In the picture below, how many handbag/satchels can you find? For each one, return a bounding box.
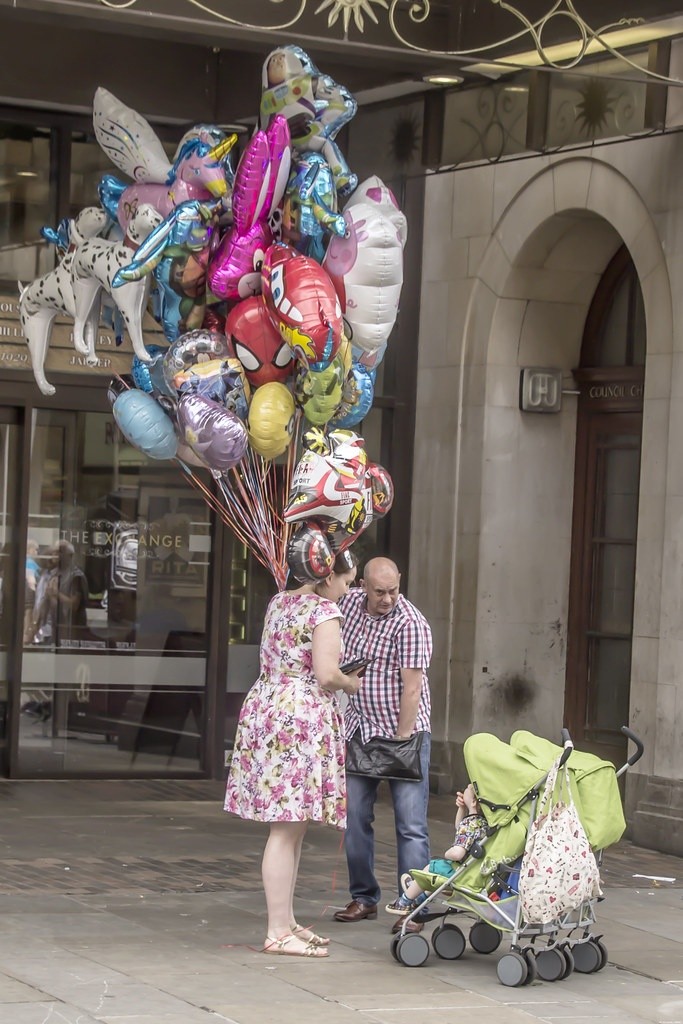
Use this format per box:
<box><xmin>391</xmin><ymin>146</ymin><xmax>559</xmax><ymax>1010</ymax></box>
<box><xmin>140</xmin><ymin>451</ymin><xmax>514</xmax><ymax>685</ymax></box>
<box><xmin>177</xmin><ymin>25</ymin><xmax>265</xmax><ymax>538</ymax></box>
<box><xmin>518</xmin><ymin>802</ymin><xmax>603</xmax><ymax>924</ymax></box>
<box><xmin>345</xmin><ymin>731</ymin><xmax>424</xmax><ymax>783</ymax></box>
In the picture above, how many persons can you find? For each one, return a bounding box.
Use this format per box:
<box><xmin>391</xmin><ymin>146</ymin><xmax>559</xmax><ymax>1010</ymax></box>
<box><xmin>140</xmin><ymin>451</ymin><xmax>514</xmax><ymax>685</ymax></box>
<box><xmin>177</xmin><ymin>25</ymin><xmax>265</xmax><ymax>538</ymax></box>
<box><xmin>334</xmin><ymin>557</ymin><xmax>431</xmax><ymax>933</ymax></box>
<box><xmin>25</xmin><ymin>538</ymin><xmax>88</xmax><ymax>716</ymax></box>
<box><xmin>223</xmin><ymin>549</ymin><xmax>365</xmax><ymax>957</ymax></box>
<box><xmin>385</xmin><ymin>784</ymin><xmax>495</xmax><ymax>914</ymax></box>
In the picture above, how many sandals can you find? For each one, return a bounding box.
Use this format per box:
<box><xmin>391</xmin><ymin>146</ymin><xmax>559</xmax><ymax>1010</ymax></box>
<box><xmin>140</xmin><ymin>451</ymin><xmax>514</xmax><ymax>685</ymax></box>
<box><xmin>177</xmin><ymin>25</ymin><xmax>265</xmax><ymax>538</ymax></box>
<box><xmin>264</xmin><ymin>924</ymin><xmax>332</xmax><ymax>956</ymax></box>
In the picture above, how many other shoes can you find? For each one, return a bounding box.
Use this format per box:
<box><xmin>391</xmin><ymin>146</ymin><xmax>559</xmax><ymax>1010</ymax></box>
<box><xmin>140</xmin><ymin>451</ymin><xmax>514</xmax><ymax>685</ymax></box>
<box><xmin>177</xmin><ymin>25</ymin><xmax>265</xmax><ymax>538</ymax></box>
<box><xmin>400</xmin><ymin>873</ymin><xmax>423</xmax><ymax>908</ymax></box>
<box><xmin>385</xmin><ymin>898</ymin><xmax>413</xmax><ymax>915</ymax></box>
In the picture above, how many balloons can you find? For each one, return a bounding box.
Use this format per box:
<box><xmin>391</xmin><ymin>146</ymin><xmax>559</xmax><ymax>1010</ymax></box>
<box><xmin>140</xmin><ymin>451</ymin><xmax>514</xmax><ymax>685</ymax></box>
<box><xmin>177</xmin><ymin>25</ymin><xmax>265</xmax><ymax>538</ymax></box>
<box><xmin>19</xmin><ymin>48</ymin><xmax>407</xmax><ymax>582</ymax></box>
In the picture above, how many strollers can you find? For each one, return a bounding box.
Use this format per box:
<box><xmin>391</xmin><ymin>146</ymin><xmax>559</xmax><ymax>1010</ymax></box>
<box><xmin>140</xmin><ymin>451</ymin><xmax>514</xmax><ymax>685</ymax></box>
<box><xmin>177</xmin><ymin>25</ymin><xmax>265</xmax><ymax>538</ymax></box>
<box><xmin>385</xmin><ymin>726</ymin><xmax>644</xmax><ymax>988</ymax></box>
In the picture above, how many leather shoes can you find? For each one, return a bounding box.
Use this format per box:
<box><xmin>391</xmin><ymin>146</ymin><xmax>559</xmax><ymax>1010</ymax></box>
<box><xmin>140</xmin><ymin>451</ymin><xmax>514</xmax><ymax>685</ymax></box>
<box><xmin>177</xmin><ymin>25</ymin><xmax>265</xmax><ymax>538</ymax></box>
<box><xmin>392</xmin><ymin>915</ymin><xmax>424</xmax><ymax>932</ymax></box>
<box><xmin>334</xmin><ymin>900</ymin><xmax>377</xmax><ymax>922</ymax></box>
